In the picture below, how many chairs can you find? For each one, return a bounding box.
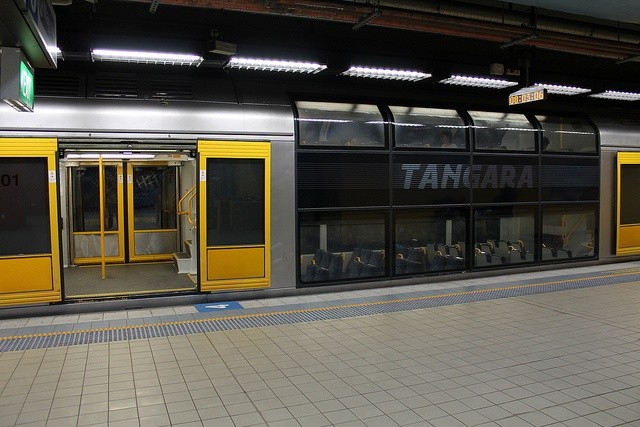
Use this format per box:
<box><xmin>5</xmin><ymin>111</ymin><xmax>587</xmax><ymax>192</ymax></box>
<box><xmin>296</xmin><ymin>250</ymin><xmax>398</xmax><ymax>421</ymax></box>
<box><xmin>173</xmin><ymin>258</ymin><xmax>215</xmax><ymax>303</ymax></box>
<box><xmin>509</xmin><ymin>240</ymin><xmax>525</xmax><ymax>263</ymax></box>
<box><xmin>425</xmin><ymin>242</ymin><xmax>442</xmax><ymax>272</ymax></box>
<box><xmin>303</xmin><ymin>250</ymin><xmax>343</xmax><ymax>282</ymax></box>
<box><xmin>498</xmin><ymin>240</ymin><xmax>507</xmax><ymax>256</ymax></box>
<box><xmin>475</xmin><ymin>241</ymin><xmax>494</xmax><ymax>268</ymax></box>
<box><xmin>347</xmin><ymin>247</ymin><xmax>384</xmax><ymax>279</ymax></box>
<box><xmin>394</xmin><ymin>254</ymin><xmax>425</xmax><ymax>274</ymax></box>
<box><xmin>443</xmin><ymin>243</ymin><xmax>465</xmax><ymax>272</ymax></box>
<box><xmin>542</xmin><ymin>247</ymin><xmax>571</xmax><ymax>260</ymax></box>
